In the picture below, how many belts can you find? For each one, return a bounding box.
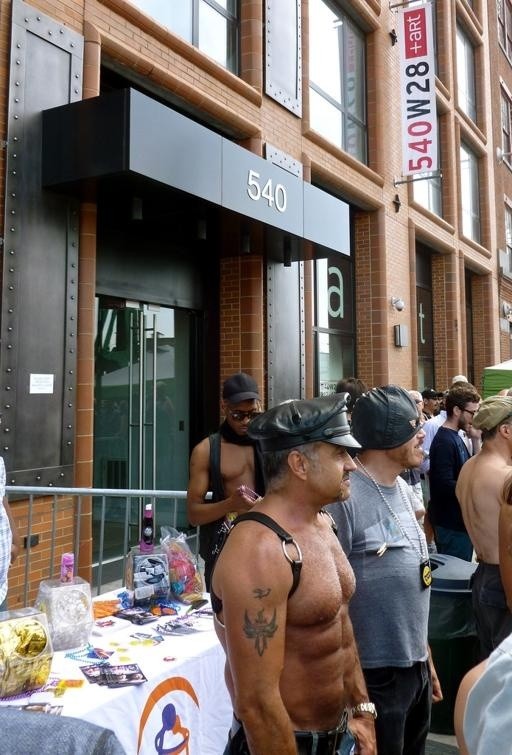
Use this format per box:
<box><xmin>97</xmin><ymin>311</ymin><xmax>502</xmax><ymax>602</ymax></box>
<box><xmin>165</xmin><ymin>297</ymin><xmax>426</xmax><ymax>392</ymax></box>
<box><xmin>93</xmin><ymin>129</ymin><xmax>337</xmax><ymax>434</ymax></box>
<box><xmin>233</xmin><ymin>708</ymin><xmax>348</xmax><ymax>755</ymax></box>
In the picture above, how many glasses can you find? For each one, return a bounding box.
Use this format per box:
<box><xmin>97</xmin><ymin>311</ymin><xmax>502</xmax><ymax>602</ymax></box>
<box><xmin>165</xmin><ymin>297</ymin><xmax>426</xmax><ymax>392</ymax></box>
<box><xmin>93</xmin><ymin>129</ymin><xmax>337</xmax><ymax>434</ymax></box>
<box><xmin>225</xmin><ymin>405</ymin><xmax>261</xmax><ymax>421</ymax></box>
<box><xmin>458</xmin><ymin>408</ymin><xmax>476</xmax><ymax>416</ymax></box>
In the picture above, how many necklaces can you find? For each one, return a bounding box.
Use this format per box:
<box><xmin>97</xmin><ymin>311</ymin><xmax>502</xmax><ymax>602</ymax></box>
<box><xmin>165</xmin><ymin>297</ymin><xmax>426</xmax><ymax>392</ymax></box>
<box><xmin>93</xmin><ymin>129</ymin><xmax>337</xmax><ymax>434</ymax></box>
<box><xmin>355</xmin><ymin>456</ymin><xmax>428</xmax><ymax>560</ymax></box>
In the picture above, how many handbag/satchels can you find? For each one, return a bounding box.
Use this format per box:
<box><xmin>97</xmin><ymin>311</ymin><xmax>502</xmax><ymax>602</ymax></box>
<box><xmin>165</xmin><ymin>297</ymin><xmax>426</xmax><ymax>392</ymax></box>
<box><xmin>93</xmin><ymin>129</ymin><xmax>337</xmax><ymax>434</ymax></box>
<box><xmin>160</xmin><ymin>525</ymin><xmax>205</xmax><ymax>605</ymax></box>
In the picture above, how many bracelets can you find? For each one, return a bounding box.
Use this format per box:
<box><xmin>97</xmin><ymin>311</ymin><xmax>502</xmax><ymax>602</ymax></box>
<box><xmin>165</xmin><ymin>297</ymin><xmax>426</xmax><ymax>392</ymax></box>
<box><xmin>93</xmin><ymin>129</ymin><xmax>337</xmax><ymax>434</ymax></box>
<box><xmin>351</xmin><ymin>702</ymin><xmax>378</xmax><ymax>719</ymax></box>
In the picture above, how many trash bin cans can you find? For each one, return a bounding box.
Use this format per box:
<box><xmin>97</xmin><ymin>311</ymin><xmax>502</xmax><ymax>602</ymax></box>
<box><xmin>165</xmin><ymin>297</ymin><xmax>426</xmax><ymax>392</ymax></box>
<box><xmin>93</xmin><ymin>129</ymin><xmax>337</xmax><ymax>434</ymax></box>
<box><xmin>428</xmin><ymin>552</ymin><xmax>483</xmax><ymax>736</ymax></box>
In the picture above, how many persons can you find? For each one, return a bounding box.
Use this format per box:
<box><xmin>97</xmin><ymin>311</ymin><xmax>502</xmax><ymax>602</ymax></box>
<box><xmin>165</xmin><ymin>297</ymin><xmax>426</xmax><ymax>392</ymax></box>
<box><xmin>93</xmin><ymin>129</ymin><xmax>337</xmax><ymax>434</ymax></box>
<box><xmin>187</xmin><ymin>373</ymin><xmax>266</xmax><ymax>592</ymax></box>
<box><xmin>322</xmin><ymin>385</ymin><xmax>444</xmax><ymax>755</ymax></box>
<box><xmin>116</xmin><ymin>674</ymin><xmax>143</xmax><ymax>683</ymax></box>
<box><xmin>336</xmin><ymin>377</ymin><xmax>368</xmax><ymax>424</ymax></box>
<box><xmin>407</xmin><ymin>375</ymin><xmax>512</xmax><ymax>755</ymax></box>
<box><xmin>0</xmin><ymin>456</ymin><xmax>19</xmax><ymax>612</ymax></box>
<box><xmin>210</xmin><ymin>391</ymin><xmax>379</xmax><ymax>755</ymax></box>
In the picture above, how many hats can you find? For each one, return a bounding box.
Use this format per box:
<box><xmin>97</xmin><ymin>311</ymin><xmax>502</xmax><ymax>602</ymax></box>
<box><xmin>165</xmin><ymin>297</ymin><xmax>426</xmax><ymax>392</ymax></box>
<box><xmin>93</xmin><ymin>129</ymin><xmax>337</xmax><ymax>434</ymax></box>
<box><xmin>421</xmin><ymin>388</ymin><xmax>443</xmax><ymax>397</ymax></box>
<box><xmin>352</xmin><ymin>384</ymin><xmax>423</xmax><ymax>449</ymax></box>
<box><xmin>473</xmin><ymin>396</ymin><xmax>511</xmax><ymax>432</ymax></box>
<box><xmin>222</xmin><ymin>373</ymin><xmax>259</xmax><ymax>404</ymax></box>
<box><xmin>247</xmin><ymin>392</ymin><xmax>363</xmax><ymax>450</ymax></box>
